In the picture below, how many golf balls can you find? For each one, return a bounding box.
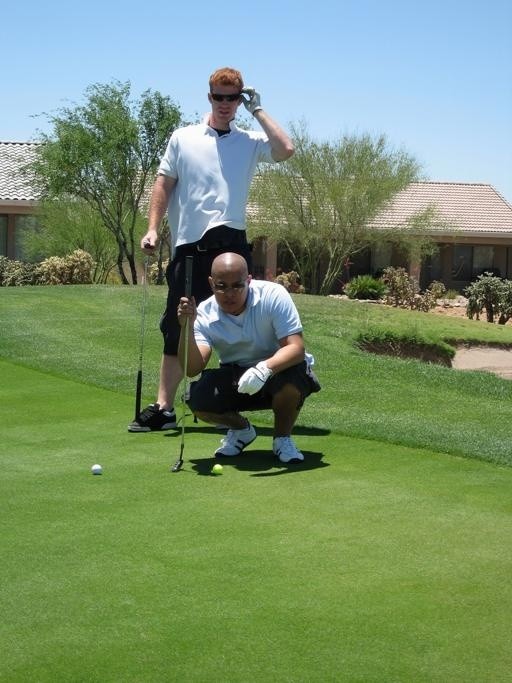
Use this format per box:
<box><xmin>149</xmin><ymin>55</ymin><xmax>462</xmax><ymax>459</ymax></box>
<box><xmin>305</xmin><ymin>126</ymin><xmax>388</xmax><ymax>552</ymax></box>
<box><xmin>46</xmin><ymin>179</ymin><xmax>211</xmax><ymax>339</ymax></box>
<box><xmin>212</xmin><ymin>464</ymin><xmax>224</xmax><ymax>475</ymax></box>
<box><xmin>91</xmin><ymin>463</ymin><xmax>101</xmax><ymax>473</ymax></box>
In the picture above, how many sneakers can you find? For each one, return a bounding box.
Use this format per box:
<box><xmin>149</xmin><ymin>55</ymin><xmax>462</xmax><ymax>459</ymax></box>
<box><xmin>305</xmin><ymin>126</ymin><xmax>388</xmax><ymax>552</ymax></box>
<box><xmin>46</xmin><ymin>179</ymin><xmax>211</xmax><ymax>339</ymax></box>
<box><xmin>214</xmin><ymin>418</ymin><xmax>257</xmax><ymax>457</ymax></box>
<box><xmin>128</xmin><ymin>402</ymin><xmax>177</xmax><ymax>432</ymax></box>
<box><xmin>273</xmin><ymin>436</ymin><xmax>305</xmax><ymax>464</ymax></box>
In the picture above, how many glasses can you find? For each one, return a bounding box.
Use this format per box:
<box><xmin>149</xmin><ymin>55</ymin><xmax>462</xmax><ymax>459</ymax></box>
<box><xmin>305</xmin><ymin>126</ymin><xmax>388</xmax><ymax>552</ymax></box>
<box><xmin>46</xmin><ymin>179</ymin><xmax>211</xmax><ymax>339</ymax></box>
<box><xmin>211</xmin><ymin>278</ymin><xmax>249</xmax><ymax>294</ymax></box>
<box><xmin>210</xmin><ymin>90</ymin><xmax>241</xmax><ymax>101</ymax></box>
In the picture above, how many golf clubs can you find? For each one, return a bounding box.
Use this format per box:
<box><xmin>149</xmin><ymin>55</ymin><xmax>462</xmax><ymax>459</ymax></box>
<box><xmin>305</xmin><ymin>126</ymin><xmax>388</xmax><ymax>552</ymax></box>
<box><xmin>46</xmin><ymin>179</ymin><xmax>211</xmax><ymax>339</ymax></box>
<box><xmin>135</xmin><ymin>240</ymin><xmax>149</xmax><ymax>417</ymax></box>
<box><xmin>172</xmin><ymin>255</ymin><xmax>193</xmax><ymax>474</ymax></box>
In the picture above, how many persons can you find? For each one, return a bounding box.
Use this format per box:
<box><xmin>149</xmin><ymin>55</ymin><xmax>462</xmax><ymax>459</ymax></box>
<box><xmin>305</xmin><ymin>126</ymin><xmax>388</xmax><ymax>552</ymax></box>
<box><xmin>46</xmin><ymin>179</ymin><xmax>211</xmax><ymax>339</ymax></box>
<box><xmin>125</xmin><ymin>66</ymin><xmax>296</xmax><ymax>432</ymax></box>
<box><xmin>178</xmin><ymin>251</ymin><xmax>321</xmax><ymax>464</ymax></box>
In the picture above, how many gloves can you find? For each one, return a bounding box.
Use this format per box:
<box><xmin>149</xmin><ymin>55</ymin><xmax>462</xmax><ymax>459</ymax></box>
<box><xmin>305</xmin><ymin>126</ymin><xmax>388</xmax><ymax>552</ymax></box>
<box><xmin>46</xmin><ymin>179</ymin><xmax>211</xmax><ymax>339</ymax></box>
<box><xmin>237</xmin><ymin>361</ymin><xmax>273</xmax><ymax>395</ymax></box>
<box><xmin>241</xmin><ymin>87</ymin><xmax>264</xmax><ymax>116</ymax></box>
